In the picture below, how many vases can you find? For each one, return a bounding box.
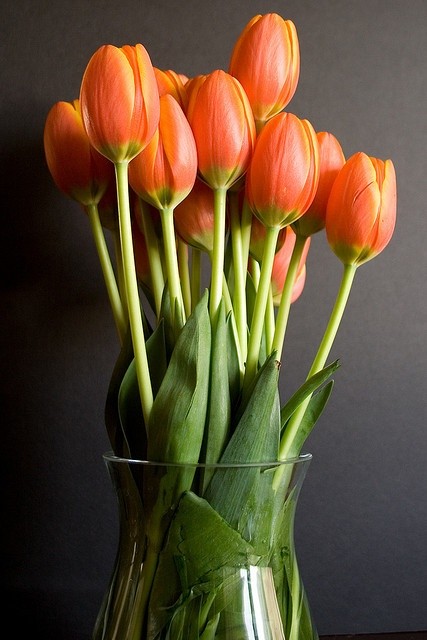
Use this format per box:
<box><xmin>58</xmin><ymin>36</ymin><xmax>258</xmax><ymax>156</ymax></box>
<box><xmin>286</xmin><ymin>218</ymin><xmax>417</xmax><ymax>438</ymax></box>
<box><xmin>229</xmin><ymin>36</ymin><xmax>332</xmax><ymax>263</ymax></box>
<box><xmin>90</xmin><ymin>451</ymin><xmax>320</xmax><ymax>640</ymax></box>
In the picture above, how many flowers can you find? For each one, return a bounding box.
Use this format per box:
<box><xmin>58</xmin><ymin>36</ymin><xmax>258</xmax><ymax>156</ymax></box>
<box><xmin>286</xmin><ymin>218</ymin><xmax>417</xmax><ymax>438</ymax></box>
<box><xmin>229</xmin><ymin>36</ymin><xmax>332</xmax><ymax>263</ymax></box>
<box><xmin>43</xmin><ymin>12</ymin><xmax>397</xmax><ymax>640</ymax></box>
